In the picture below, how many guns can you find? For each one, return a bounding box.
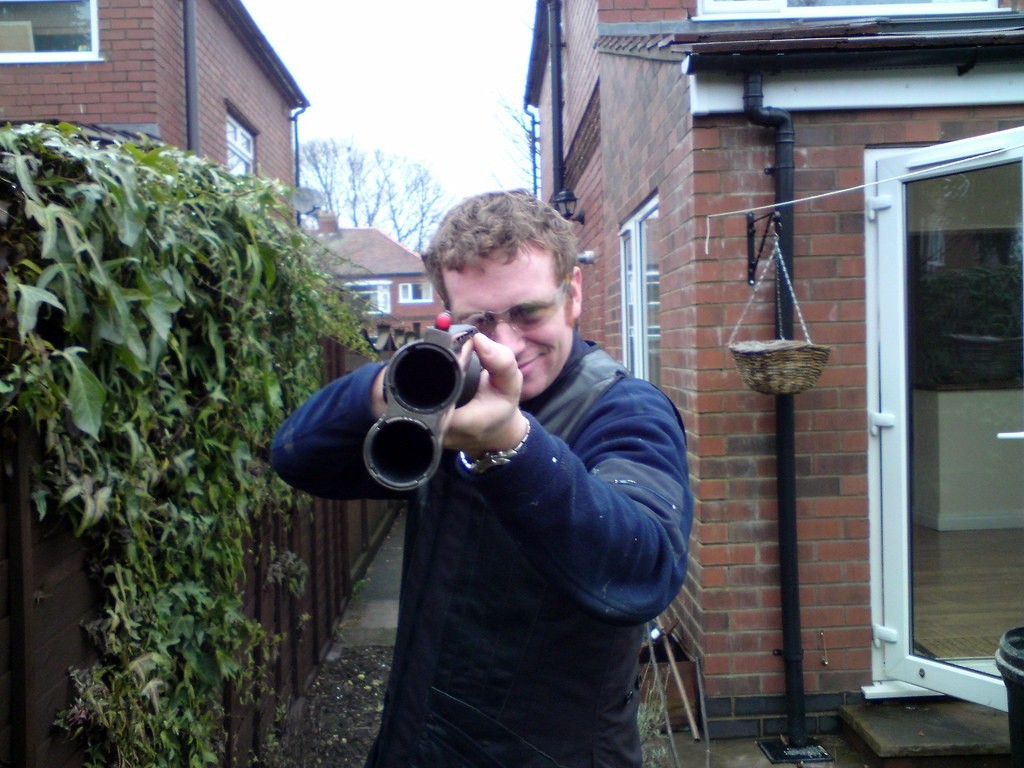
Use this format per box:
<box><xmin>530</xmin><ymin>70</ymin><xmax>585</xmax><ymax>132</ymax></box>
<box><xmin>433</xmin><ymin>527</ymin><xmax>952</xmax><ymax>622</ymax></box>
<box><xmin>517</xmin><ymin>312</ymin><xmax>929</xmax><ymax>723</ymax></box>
<box><xmin>365</xmin><ymin>315</ymin><xmax>483</xmax><ymax>488</ymax></box>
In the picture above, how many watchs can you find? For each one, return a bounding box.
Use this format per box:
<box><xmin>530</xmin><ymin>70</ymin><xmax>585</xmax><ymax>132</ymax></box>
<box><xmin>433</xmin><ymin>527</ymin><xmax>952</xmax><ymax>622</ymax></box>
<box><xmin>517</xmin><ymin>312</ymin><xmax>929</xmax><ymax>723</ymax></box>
<box><xmin>458</xmin><ymin>417</ymin><xmax>530</xmax><ymax>473</ymax></box>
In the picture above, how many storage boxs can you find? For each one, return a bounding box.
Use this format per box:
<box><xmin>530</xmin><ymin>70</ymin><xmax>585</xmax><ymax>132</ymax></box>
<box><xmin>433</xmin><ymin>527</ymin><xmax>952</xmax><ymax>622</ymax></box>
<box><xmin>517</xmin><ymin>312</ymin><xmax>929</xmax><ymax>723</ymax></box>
<box><xmin>638</xmin><ymin>616</ymin><xmax>699</xmax><ymax>733</ymax></box>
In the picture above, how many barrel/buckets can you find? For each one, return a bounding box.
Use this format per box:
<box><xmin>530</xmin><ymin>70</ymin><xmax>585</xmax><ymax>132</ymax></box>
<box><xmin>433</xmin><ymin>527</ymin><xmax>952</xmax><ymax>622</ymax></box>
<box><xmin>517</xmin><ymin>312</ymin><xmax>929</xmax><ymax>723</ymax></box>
<box><xmin>994</xmin><ymin>625</ymin><xmax>1024</xmax><ymax>768</ymax></box>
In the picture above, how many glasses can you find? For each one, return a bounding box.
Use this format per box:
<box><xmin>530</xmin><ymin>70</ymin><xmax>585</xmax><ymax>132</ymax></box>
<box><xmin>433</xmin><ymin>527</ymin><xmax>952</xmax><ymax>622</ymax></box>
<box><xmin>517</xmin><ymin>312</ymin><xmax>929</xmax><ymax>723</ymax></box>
<box><xmin>445</xmin><ymin>279</ymin><xmax>567</xmax><ymax>341</ymax></box>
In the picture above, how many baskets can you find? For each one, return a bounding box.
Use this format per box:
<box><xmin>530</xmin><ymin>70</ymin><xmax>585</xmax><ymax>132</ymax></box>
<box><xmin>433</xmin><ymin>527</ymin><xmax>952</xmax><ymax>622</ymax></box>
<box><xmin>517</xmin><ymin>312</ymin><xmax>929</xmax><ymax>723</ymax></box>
<box><xmin>728</xmin><ymin>341</ymin><xmax>831</xmax><ymax>395</ymax></box>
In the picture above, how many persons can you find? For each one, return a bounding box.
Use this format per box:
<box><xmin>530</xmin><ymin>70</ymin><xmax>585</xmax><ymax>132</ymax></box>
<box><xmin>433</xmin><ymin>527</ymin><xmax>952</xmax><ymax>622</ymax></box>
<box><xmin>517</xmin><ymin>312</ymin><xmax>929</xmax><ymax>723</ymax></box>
<box><xmin>269</xmin><ymin>189</ymin><xmax>697</xmax><ymax>768</ymax></box>
